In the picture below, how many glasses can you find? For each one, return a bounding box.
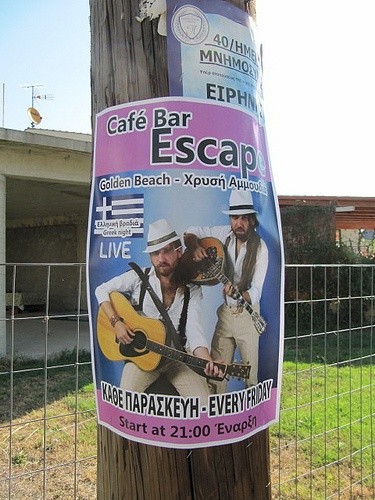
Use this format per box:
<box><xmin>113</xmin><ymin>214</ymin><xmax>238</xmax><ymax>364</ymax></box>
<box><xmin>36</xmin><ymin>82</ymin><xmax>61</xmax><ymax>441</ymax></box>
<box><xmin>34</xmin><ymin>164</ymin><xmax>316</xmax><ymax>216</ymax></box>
<box><xmin>149</xmin><ymin>246</ymin><xmax>182</xmax><ymax>256</ymax></box>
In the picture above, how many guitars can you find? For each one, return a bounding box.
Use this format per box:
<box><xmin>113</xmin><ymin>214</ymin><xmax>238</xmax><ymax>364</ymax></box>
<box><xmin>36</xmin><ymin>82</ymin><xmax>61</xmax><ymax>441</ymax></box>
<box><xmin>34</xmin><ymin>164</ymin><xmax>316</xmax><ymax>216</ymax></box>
<box><xmin>180</xmin><ymin>236</ymin><xmax>267</xmax><ymax>336</ymax></box>
<box><xmin>96</xmin><ymin>291</ymin><xmax>251</xmax><ymax>381</ymax></box>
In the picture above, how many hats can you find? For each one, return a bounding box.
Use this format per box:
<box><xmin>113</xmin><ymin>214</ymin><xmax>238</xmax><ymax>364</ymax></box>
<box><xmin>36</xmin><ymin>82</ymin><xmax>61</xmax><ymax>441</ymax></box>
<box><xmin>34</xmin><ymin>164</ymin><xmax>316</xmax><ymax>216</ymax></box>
<box><xmin>222</xmin><ymin>190</ymin><xmax>258</xmax><ymax>216</ymax></box>
<box><xmin>141</xmin><ymin>219</ymin><xmax>183</xmax><ymax>253</ymax></box>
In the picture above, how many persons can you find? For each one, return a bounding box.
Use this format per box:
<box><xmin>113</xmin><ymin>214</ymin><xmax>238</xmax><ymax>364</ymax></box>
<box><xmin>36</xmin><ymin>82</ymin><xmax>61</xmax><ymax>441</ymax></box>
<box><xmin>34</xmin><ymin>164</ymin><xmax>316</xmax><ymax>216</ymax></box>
<box><xmin>95</xmin><ymin>219</ymin><xmax>226</xmax><ymax>413</ymax></box>
<box><xmin>184</xmin><ymin>189</ymin><xmax>269</xmax><ymax>396</ymax></box>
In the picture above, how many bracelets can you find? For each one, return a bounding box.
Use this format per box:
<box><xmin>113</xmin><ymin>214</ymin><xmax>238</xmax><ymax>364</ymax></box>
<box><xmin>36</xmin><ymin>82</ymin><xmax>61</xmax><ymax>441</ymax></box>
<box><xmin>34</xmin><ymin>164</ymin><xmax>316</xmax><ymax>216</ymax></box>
<box><xmin>108</xmin><ymin>313</ymin><xmax>121</xmax><ymax>327</ymax></box>
